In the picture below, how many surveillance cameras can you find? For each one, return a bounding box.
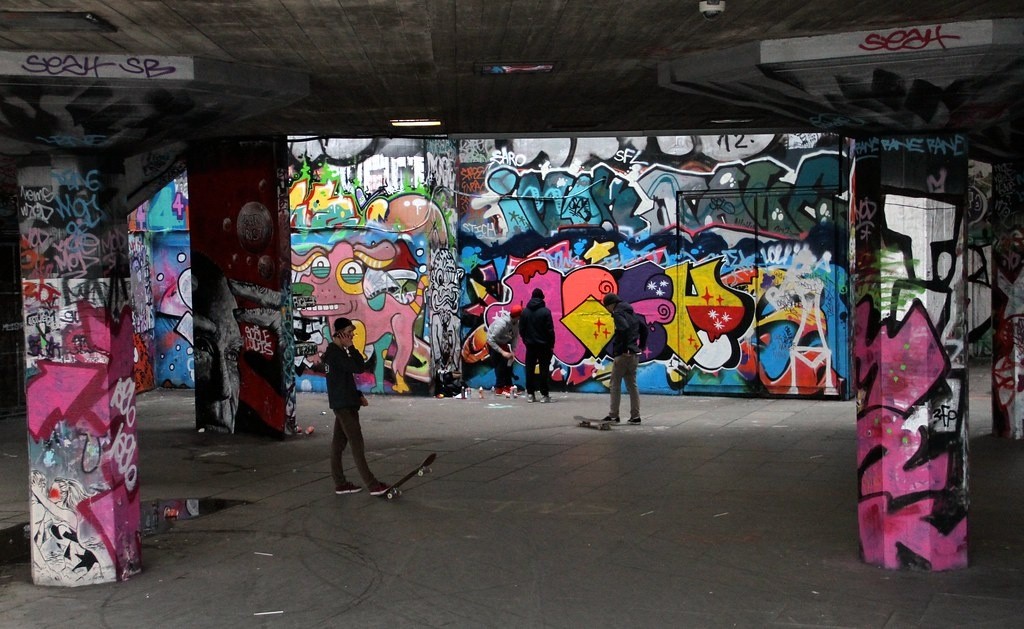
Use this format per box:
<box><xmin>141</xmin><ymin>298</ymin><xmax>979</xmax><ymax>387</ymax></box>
<box><xmin>699</xmin><ymin>0</ymin><xmax>726</xmax><ymax>21</ymax></box>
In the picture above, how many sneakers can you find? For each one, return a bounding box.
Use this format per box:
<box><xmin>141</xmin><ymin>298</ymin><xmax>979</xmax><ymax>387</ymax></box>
<box><xmin>527</xmin><ymin>395</ymin><xmax>536</xmax><ymax>403</ymax></box>
<box><xmin>368</xmin><ymin>477</ymin><xmax>391</xmax><ymax>495</ymax></box>
<box><xmin>540</xmin><ymin>394</ymin><xmax>551</xmax><ymax>402</ymax></box>
<box><xmin>496</xmin><ymin>387</ymin><xmax>518</xmax><ymax>398</ymax></box>
<box><xmin>602</xmin><ymin>415</ymin><xmax>620</xmax><ymax>422</ymax></box>
<box><xmin>627</xmin><ymin>416</ymin><xmax>641</xmax><ymax>424</ymax></box>
<box><xmin>335</xmin><ymin>480</ymin><xmax>363</xmax><ymax>494</ymax></box>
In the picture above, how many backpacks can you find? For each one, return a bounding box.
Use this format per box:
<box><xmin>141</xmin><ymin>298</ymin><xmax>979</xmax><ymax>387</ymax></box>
<box><xmin>434</xmin><ymin>370</ymin><xmax>466</xmax><ymax>397</ymax></box>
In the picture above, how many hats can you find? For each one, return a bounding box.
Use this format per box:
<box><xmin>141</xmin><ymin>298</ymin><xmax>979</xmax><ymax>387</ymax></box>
<box><xmin>531</xmin><ymin>287</ymin><xmax>545</xmax><ymax>299</ymax></box>
<box><xmin>334</xmin><ymin>317</ymin><xmax>352</xmax><ymax>331</ymax></box>
<box><xmin>603</xmin><ymin>292</ymin><xmax>622</xmax><ymax>306</ymax></box>
<box><xmin>510</xmin><ymin>303</ymin><xmax>524</xmax><ymax>314</ymax></box>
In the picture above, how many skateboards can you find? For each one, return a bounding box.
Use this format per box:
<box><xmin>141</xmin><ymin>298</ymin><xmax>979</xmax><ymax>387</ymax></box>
<box><xmin>380</xmin><ymin>451</ymin><xmax>437</xmax><ymax>499</ymax></box>
<box><xmin>573</xmin><ymin>414</ymin><xmax>619</xmax><ymax>429</ymax></box>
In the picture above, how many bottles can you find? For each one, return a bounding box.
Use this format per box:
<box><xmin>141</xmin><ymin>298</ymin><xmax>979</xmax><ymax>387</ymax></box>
<box><xmin>510</xmin><ymin>387</ymin><xmax>514</xmax><ymax>399</ymax></box>
<box><xmin>467</xmin><ymin>387</ymin><xmax>471</xmax><ymax>399</ymax></box>
<box><xmin>461</xmin><ymin>386</ymin><xmax>465</xmax><ymax>398</ymax></box>
<box><xmin>491</xmin><ymin>385</ymin><xmax>495</xmax><ymax>394</ymax></box>
<box><xmin>479</xmin><ymin>385</ymin><xmax>484</xmax><ymax>399</ymax></box>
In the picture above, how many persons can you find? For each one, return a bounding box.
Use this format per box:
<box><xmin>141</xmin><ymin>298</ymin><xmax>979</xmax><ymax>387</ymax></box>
<box><xmin>602</xmin><ymin>292</ymin><xmax>648</xmax><ymax>425</ymax></box>
<box><xmin>319</xmin><ymin>317</ymin><xmax>392</xmax><ymax>495</ymax></box>
<box><xmin>485</xmin><ymin>305</ymin><xmax>524</xmax><ymax>397</ymax></box>
<box><xmin>520</xmin><ymin>287</ymin><xmax>558</xmax><ymax>403</ymax></box>
